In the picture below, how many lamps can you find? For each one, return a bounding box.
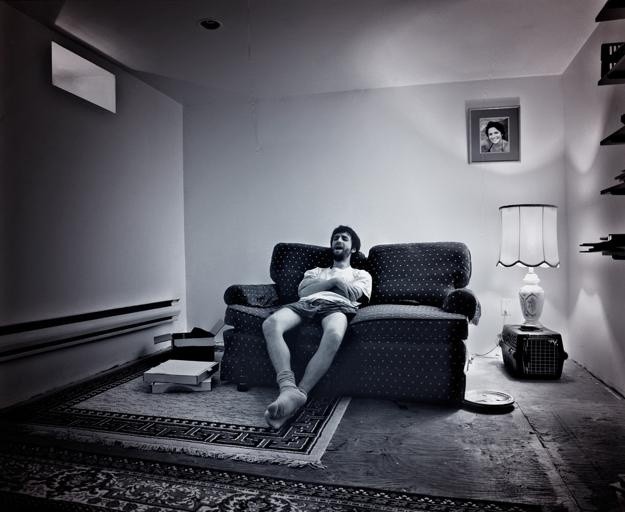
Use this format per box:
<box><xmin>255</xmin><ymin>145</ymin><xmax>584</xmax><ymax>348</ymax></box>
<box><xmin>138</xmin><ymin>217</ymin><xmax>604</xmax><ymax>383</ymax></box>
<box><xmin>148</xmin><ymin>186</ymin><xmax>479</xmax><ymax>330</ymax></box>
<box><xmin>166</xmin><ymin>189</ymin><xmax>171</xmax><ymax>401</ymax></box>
<box><xmin>494</xmin><ymin>202</ymin><xmax>562</xmax><ymax>332</ymax></box>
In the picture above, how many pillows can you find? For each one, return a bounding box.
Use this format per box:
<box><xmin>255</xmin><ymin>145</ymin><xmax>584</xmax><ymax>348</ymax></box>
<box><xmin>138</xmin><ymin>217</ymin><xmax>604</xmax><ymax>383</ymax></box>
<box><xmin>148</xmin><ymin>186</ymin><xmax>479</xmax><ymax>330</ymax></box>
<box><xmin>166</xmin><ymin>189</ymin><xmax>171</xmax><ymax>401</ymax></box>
<box><xmin>367</xmin><ymin>242</ymin><xmax>473</xmax><ymax>307</ymax></box>
<box><xmin>269</xmin><ymin>242</ymin><xmax>367</xmax><ymax>304</ymax></box>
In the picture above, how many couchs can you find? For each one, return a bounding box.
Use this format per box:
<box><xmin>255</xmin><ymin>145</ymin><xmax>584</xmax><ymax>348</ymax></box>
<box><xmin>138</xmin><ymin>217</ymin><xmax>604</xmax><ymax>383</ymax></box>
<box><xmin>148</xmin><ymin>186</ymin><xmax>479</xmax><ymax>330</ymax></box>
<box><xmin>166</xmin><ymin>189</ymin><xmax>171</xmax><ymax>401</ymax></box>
<box><xmin>220</xmin><ymin>241</ymin><xmax>482</xmax><ymax>411</ymax></box>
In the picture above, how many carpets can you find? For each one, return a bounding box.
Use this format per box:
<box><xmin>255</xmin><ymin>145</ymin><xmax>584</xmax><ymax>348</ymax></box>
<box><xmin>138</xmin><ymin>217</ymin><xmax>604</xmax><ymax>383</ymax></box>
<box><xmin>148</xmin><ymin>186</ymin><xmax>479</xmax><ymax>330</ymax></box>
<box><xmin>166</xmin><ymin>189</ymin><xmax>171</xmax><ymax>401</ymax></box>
<box><xmin>0</xmin><ymin>439</ymin><xmax>570</xmax><ymax>512</ymax></box>
<box><xmin>1</xmin><ymin>346</ymin><xmax>351</xmax><ymax>470</ymax></box>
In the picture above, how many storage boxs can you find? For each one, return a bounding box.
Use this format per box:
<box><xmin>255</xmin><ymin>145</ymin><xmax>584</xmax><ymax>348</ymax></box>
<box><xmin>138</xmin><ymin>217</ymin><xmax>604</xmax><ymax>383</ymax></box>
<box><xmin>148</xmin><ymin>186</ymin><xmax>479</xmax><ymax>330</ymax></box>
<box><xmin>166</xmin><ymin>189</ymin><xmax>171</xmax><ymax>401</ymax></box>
<box><xmin>153</xmin><ymin>318</ymin><xmax>226</xmax><ymax>362</ymax></box>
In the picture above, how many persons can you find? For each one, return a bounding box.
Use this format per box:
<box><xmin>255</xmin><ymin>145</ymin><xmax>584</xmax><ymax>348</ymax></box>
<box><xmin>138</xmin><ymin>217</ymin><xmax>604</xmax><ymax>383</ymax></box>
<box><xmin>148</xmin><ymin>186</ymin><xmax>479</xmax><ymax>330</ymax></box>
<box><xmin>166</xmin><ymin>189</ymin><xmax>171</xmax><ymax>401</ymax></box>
<box><xmin>485</xmin><ymin>121</ymin><xmax>509</xmax><ymax>153</ymax></box>
<box><xmin>260</xmin><ymin>225</ymin><xmax>375</xmax><ymax>431</ymax></box>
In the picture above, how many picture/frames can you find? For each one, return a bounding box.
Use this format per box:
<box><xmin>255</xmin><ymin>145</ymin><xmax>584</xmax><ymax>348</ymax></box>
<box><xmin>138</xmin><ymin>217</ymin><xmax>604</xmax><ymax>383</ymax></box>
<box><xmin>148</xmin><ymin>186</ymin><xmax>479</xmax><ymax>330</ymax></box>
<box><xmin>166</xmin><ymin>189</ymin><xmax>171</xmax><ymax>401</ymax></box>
<box><xmin>466</xmin><ymin>104</ymin><xmax>521</xmax><ymax>165</ymax></box>
